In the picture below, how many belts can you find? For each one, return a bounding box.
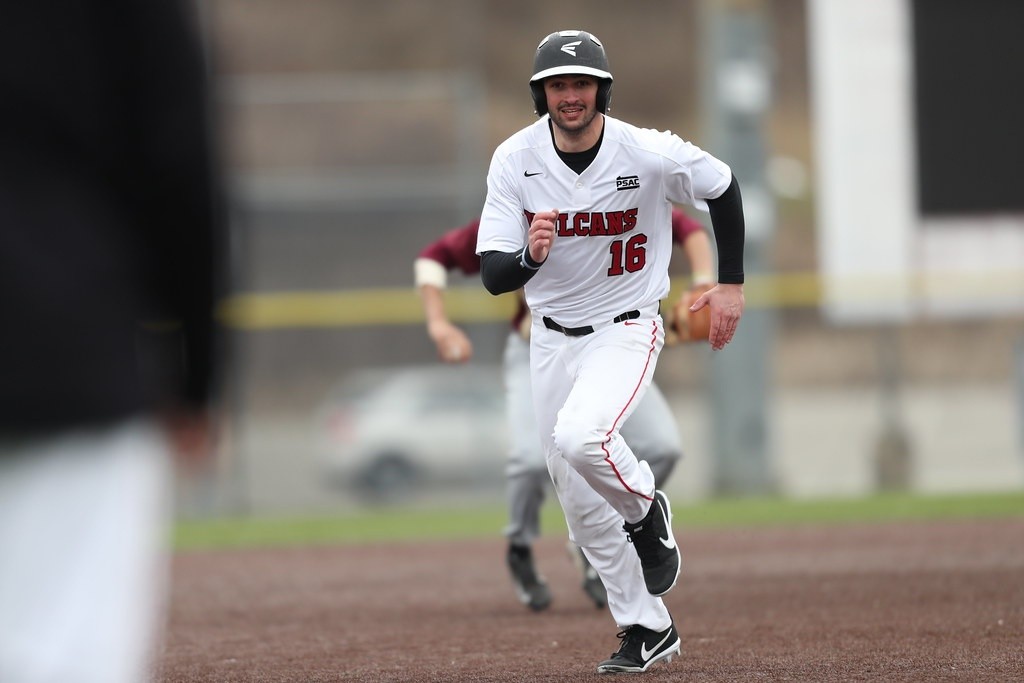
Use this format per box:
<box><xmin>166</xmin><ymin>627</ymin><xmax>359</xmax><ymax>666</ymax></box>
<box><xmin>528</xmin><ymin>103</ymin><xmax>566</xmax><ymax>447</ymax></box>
<box><xmin>543</xmin><ymin>310</ymin><xmax>639</xmax><ymax>337</ymax></box>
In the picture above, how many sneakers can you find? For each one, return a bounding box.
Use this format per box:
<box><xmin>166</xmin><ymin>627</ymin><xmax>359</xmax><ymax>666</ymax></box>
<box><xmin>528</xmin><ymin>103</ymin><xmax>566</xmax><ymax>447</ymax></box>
<box><xmin>621</xmin><ymin>489</ymin><xmax>681</xmax><ymax>597</ymax></box>
<box><xmin>506</xmin><ymin>542</ymin><xmax>552</xmax><ymax>611</ymax></box>
<box><xmin>596</xmin><ymin>612</ymin><xmax>681</xmax><ymax>675</ymax></box>
<box><xmin>565</xmin><ymin>539</ymin><xmax>607</xmax><ymax>607</ymax></box>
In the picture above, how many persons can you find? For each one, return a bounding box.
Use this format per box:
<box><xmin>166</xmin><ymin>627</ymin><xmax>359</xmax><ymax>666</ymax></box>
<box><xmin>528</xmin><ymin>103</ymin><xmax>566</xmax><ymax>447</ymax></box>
<box><xmin>0</xmin><ymin>0</ymin><xmax>229</xmax><ymax>683</ymax></box>
<box><xmin>415</xmin><ymin>29</ymin><xmax>743</xmax><ymax>674</ymax></box>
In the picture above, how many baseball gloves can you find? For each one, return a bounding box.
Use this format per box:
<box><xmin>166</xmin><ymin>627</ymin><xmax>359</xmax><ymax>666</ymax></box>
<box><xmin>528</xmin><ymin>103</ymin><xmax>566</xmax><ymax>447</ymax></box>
<box><xmin>659</xmin><ymin>285</ymin><xmax>717</xmax><ymax>344</ymax></box>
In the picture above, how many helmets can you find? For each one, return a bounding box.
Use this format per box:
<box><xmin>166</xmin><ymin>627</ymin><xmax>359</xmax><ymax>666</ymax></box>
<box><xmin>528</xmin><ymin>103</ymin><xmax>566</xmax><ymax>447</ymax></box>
<box><xmin>529</xmin><ymin>29</ymin><xmax>613</xmax><ymax>118</ymax></box>
